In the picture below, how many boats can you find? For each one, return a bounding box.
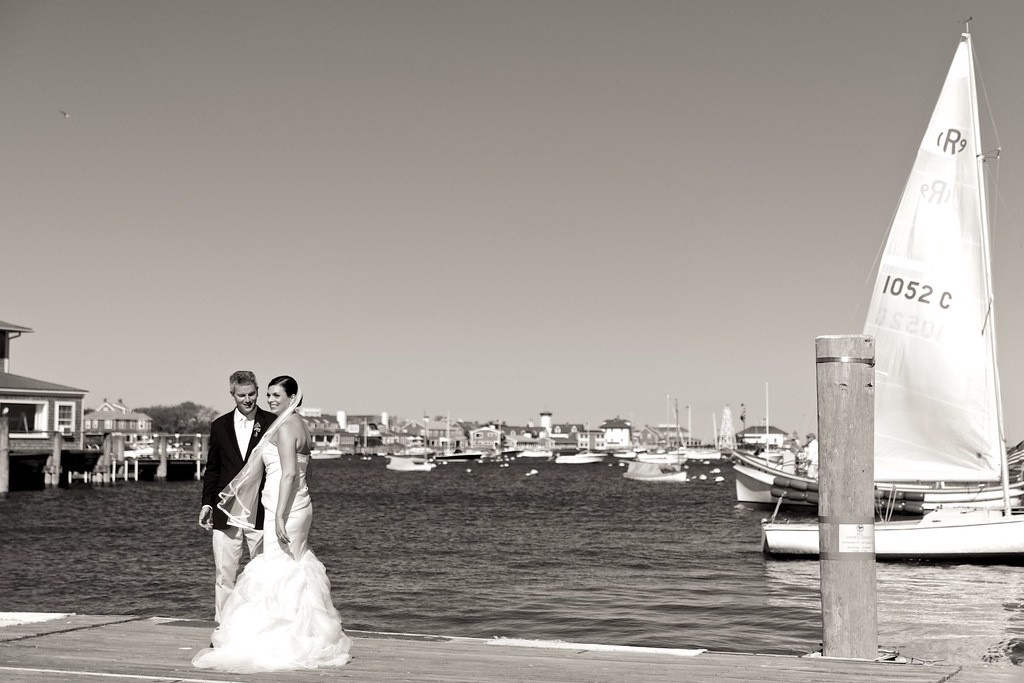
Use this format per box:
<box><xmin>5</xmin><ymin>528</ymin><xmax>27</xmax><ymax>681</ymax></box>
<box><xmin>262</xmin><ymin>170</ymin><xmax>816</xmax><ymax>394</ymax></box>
<box><xmin>386</xmin><ymin>381</ymin><xmax>1024</xmax><ymax>515</ymax></box>
<box><xmin>310</xmin><ymin>447</ymin><xmax>342</xmax><ymax>460</ymax></box>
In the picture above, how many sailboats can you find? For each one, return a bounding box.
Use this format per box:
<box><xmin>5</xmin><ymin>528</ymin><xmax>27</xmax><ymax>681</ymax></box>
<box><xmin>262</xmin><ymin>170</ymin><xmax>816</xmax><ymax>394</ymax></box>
<box><xmin>759</xmin><ymin>18</ymin><xmax>1024</xmax><ymax>565</ymax></box>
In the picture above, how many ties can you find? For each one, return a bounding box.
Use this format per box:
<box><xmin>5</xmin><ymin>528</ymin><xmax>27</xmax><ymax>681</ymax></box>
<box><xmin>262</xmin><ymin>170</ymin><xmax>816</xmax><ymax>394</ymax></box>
<box><xmin>241</xmin><ymin>417</ymin><xmax>248</xmax><ymax>435</ymax></box>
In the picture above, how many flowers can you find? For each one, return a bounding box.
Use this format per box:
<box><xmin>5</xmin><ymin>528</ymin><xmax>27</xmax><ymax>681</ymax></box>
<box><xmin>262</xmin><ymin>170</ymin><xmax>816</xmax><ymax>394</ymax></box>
<box><xmin>252</xmin><ymin>423</ymin><xmax>261</xmax><ymax>436</ymax></box>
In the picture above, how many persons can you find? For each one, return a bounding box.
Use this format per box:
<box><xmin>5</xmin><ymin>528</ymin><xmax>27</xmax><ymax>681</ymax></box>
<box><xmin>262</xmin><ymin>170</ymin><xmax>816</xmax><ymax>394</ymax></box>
<box><xmin>192</xmin><ymin>375</ymin><xmax>352</xmax><ymax>673</ymax></box>
<box><xmin>804</xmin><ymin>433</ymin><xmax>820</xmax><ymax>479</ymax></box>
<box><xmin>198</xmin><ymin>370</ymin><xmax>278</xmax><ymax>648</ymax></box>
